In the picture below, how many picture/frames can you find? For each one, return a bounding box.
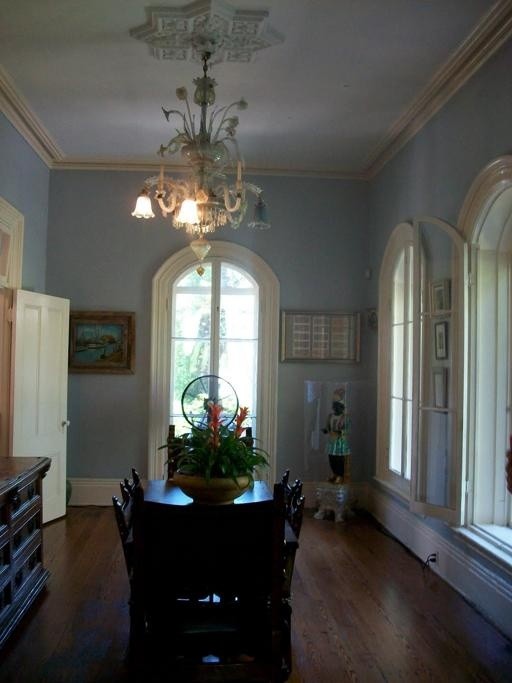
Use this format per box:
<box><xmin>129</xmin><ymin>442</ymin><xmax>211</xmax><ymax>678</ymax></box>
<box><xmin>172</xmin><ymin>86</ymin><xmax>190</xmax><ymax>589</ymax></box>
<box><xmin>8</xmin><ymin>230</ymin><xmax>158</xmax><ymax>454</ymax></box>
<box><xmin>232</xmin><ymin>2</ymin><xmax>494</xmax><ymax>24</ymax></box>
<box><xmin>68</xmin><ymin>310</ymin><xmax>136</xmax><ymax>375</ymax></box>
<box><xmin>428</xmin><ymin>278</ymin><xmax>452</xmax><ymax>415</ymax></box>
<box><xmin>282</xmin><ymin>311</ymin><xmax>362</xmax><ymax>365</ymax></box>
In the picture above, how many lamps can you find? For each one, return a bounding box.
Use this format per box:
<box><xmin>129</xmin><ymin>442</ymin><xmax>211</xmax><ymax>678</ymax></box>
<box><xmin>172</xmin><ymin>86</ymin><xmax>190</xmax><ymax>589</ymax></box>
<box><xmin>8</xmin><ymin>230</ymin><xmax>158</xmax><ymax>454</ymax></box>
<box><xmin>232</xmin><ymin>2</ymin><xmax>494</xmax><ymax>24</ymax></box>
<box><xmin>129</xmin><ymin>32</ymin><xmax>271</xmax><ymax>279</ymax></box>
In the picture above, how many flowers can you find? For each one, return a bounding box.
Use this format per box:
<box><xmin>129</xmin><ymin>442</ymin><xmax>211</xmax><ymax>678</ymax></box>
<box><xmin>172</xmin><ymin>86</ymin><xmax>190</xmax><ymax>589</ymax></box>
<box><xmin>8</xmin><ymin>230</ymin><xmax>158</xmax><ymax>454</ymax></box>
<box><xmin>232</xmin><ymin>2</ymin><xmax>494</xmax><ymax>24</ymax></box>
<box><xmin>156</xmin><ymin>403</ymin><xmax>273</xmax><ymax>494</ymax></box>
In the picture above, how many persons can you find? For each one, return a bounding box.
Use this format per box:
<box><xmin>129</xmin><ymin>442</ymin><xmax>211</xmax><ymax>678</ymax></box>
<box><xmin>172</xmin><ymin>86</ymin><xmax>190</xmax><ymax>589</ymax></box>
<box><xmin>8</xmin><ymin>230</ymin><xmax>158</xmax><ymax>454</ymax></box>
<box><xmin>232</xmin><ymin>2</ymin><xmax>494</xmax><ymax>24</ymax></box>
<box><xmin>319</xmin><ymin>387</ymin><xmax>352</xmax><ymax>484</ymax></box>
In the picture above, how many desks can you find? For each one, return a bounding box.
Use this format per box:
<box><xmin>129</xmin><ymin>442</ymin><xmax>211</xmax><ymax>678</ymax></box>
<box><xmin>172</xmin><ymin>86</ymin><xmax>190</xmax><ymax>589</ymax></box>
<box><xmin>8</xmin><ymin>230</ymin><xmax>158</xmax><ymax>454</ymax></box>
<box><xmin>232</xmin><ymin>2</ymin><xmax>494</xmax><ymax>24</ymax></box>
<box><xmin>143</xmin><ymin>479</ymin><xmax>277</xmax><ymax>528</ymax></box>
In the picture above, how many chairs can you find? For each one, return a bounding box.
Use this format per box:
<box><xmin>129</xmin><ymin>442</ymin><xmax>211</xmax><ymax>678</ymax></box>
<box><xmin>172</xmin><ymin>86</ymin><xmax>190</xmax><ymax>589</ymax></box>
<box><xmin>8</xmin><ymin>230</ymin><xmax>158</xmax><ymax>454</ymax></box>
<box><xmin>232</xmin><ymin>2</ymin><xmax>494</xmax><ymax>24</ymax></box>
<box><xmin>111</xmin><ymin>481</ymin><xmax>212</xmax><ymax>634</ymax></box>
<box><xmin>279</xmin><ymin>483</ymin><xmax>306</xmax><ymax>568</ymax></box>
<box><xmin>130</xmin><ymin>479</ymin><xmax>288</xmax><ymax>682</ymax></box>
<box><xmin>276</xmin><ymin>466</ymin><xmax>289</xmax><ymax>485</ymax></box>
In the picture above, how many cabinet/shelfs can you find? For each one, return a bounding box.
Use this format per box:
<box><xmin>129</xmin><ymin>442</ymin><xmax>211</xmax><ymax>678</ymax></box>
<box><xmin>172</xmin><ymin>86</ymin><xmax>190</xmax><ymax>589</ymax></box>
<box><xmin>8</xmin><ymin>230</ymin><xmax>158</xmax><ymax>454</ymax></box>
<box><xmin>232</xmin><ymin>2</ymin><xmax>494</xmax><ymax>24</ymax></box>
<box><xmin>0</xmin><ymin>454</ymin><xmax>53</xmax><ymax>649</ymax></box>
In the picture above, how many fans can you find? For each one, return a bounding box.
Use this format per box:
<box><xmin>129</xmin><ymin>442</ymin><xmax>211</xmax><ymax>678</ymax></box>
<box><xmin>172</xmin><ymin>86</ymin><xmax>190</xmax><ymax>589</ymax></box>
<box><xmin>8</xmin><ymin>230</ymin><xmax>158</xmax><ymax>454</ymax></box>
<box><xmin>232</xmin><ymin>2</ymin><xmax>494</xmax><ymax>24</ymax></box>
<box><xmin>181</xmin><ymin>375</ymin><xmax>239</xmax><ymax>431</ymax></box>
